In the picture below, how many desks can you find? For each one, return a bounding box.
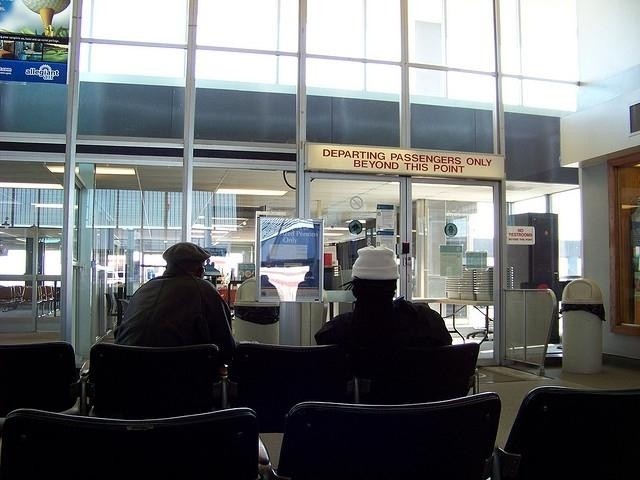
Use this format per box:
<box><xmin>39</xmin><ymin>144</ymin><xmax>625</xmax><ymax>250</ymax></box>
<box><xmin>412</xmin><ymin>297</ymin><xmax>495</xmax><ymax>348</ymax></box>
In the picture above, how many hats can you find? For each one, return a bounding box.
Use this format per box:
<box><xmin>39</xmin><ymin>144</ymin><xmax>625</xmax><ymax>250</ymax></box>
<box><xmin>352</xmin><ymin>248</ymin><xmax>399</xmax><ymax>280</ymax></box>
<box><xmin>162</xmin><ymin>243</ymin><xmax>210</xmax><ymax>261</ymax></box>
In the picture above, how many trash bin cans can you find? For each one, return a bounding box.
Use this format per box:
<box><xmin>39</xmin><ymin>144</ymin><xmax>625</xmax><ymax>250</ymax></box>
<box><xmin>234</xmin><ymin>276</ymin><xmax>280</xmax><ymax>344</ymax></box>
<box><xmin>561</xmin><ymin>278</ymin><xmax>604</xmax><ymax>374</ymax></box>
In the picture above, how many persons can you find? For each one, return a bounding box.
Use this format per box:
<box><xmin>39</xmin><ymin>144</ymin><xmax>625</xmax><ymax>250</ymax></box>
<box><xmin>211</xmin><ymin>262</ymin><xmax>220</xmax><ymax>273</ymax></box>
<box><xmin>204</xmin><ymin>259</ymin><xmax>214</xmax><ymax>272</ymax></box>
<box><xmin>261</xmin><ymin>275</ymin><xmax>275</xmax><ymax>289</ymax></box>
<box><xmin>298</xmin><ymin>272</ymin><xmax>312</xmax><ymax>287</ymax></box>
<box><xmin>314</xmin><ymin>246</ymin><xmax>453</xmax><ymax>348</ymax></box>
<box><xmin>114</xmin><ymin>243</ymin><xmax>236</xmax><ymax>367</ymax></box>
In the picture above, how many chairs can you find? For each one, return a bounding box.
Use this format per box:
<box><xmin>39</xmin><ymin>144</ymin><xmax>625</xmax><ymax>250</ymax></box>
<box><xmin>0</xmin><ymin>408</ymin><xmax>259</xmax><ymax>480</ymax></box>
<box><xmin>496</xmin><ymin>384</ymin><xmax>640</xmax><ymax>479</ymax></box>
<box><xmin>0</xmin><ymin>285</ymin><xmax>60</xmax><ymax>319</ymax></box>
<box><xmin>359</xmin><ymin>342</ymin><xmax>481</xmax><ymax>405</ymax></box>
<box><xmin>0</xmin><ymin>342</ymin><xmax>83</xmax><ymax>413</ymax></box>
<box><xmin>235</xmin><ymin>340</ymin><xmax>358</xmax><ymax>433</ymax></box>
<box><xmin>80</xmin><ymin>341</ymin><xmax>231</xmax><ymax>420</ymax></box>
<box><xmin>259</xmin><ymin>392</ymin><xmax>501</xmax><ymax>480</ymax></box>
